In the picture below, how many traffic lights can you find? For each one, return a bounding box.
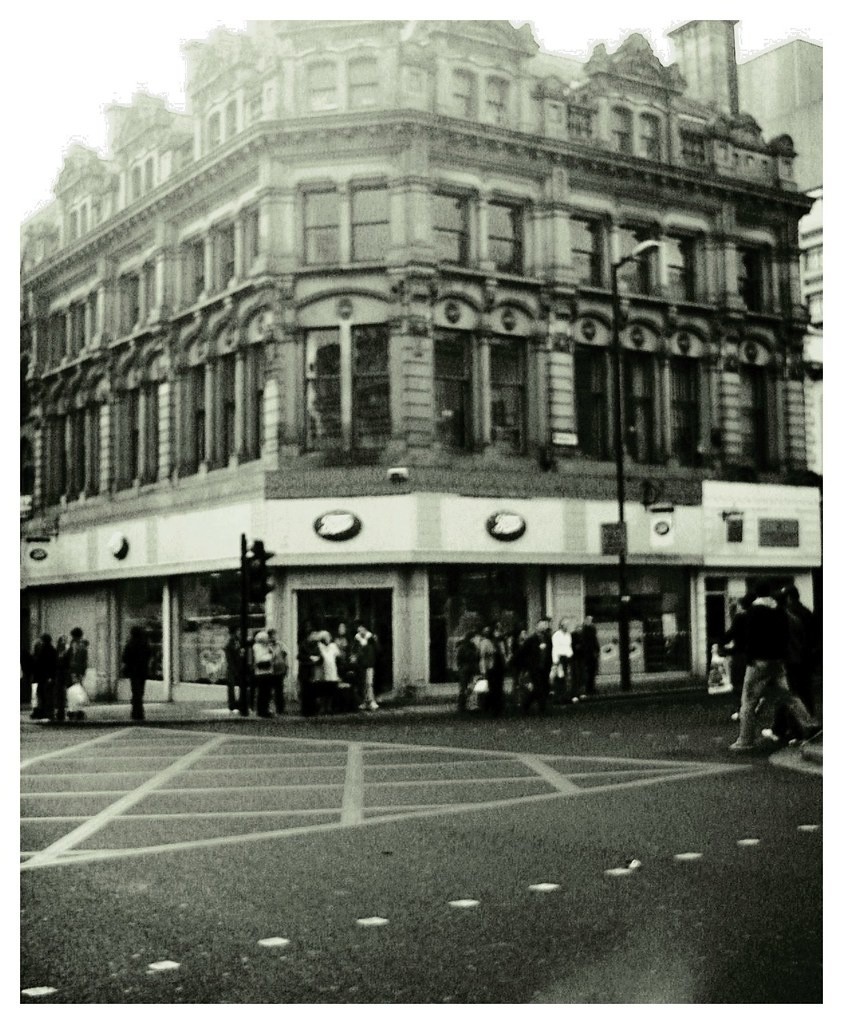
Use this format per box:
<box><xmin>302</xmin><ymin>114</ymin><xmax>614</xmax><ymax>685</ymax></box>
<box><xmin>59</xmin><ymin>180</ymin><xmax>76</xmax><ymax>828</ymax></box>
<box><xmin>245</xmin><ymin>541</ymin><xmax>279</xmax><ymax>604</ymax></box>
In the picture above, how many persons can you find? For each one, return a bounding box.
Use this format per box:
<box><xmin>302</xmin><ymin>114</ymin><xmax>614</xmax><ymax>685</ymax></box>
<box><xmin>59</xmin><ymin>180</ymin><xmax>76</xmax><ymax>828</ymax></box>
<box><xmin>453</xmin><ymin>615</ymin><xmax>601</xmax><ymax>717</ymax></box>
<box><xmin>29</xmin><ymin>627</ymin><xmax>89</xmax><ymax>721</ymax></box>
<box><xmin>298</xmin><ymin>619</ymin><xmax>380</xmax><ymax>713</ymax></box>
<box><xmin>222</xmin><ymin>628</ymin><xmax>289</xmax><ymax>718</ymax></box>
<box><xmin>718</xmin><ymin>580</ymin><xmax>823</xmax><ymax>756</ymax></box>
<box><xmin>118</xmin><ymin>626</ymin><xmax>150</xmax><ymax>720</ymax></box>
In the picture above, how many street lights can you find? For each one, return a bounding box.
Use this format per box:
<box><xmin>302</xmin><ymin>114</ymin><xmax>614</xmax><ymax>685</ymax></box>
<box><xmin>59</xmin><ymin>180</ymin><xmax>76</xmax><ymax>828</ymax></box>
<box><xmin>608</xmin><ymin>238</ymin><xmax>664</xmax><ymax>693</ymax></box>
